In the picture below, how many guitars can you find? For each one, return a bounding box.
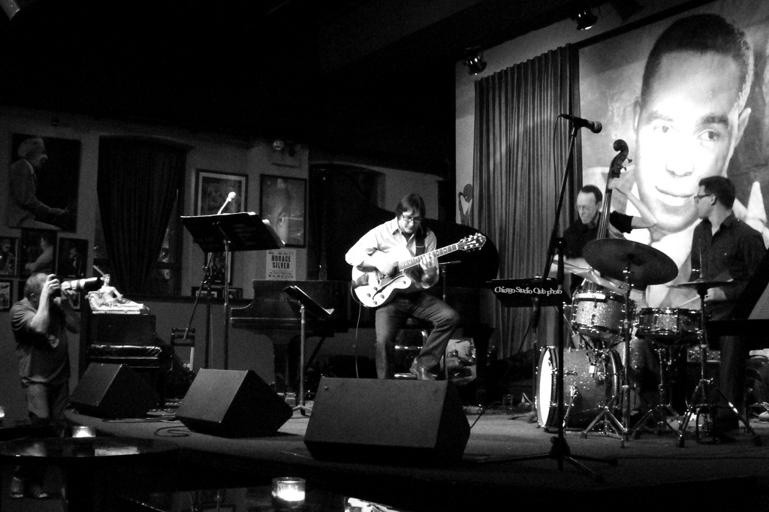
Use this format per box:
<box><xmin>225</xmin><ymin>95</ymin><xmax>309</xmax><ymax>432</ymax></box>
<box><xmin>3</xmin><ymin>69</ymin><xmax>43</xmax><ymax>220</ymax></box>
<box><xmin>351</xmin><ymin>232</ymin><xmax>487</xmax><ymax>308</ymax></box>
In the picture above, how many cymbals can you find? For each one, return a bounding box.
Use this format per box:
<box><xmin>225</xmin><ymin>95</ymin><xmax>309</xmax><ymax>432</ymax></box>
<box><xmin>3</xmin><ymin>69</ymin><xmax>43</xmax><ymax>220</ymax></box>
<box><xmin>582</xmin><ymin>238</ymin><xmax>678</xmax><ymax>285</ymax></box>
<box><xmin>665</xmin><ymin>278</ymin><xmax>734</xmax><ymax>289</ymax></box>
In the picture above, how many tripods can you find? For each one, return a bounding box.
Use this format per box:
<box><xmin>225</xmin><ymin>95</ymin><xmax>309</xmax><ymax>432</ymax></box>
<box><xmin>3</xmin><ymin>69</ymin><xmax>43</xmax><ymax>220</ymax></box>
<box><xmin>676</xmin><ymin>317</ymin><xmax>756</xmax><ymax>448</ymax></box>
<box><xmin>580</xmin><ymin>337</ymin><xmax>629</xmax><ymax>439</ymax></box>
<box><xmin>472</xmin><ymin>324</ymin><xmax>617</xmax><ymax>479</ymax></box>
<box><xmin>287</xmin><ymin>341</ymin><xmax>313</xmax><ymax>416</ymax></box>
<box><xmin>623</xmin><ymin>349</ymin><xmax>692</xmax><ymax>439</ymax></box>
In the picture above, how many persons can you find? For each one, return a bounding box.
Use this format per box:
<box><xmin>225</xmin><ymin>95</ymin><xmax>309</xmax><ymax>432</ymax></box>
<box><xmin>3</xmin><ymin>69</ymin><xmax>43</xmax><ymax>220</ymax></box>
<box><xmin>25</xmin><ymin>234</ymin><xmax>54</xmax><ymax>272</ymax></box>
<box><xmin>343</xmin><ymin>191</ymin><xmax>461</xmax><ymax>381</ymax></box>
<box><xmin>87</xmin><ymin>273</ymin><xmax>145</xmax><ymax>308</ymax></box>
<box><xmin>582</xmin><ymin>10</ymin><xmax>768</xmax><ymax>320</ymax></box>
<box><xmin>262</xmin><ymin>176</ymin><xmax>291</xmax><ymax>243</ymax></box>
<box><xmin>0</xmin><ymin>238</ymin><xmax>14</xmax><ymax>275</ymax></box>
<box><xmin>8</xmin><ymin>270</ymin><xmax>82</xmax><ymax>501</ymax></box>
<box><xmin>561</xmin><ymin>178</ymin><xmax>657</xmax><ymax>299</ymax></box>
<box><xmin>685</xmin><ymin>175</ymin><xmax>769</xmax><ymax>446</ymax></box>
<box><xmin>7</xmin><ymin>137</ymin><xmax>68</xmax><ymax>230</ymax></box>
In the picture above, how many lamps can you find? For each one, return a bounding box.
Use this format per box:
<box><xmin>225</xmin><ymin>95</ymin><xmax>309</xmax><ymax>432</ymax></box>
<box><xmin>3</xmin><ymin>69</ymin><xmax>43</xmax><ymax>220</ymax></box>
<box><xmin>457</xmin><ymin>41</ymin><xmax>489</xmax><ymax>77</ymax></box>
<box><xmin>271</xmin><ymin>134</ymin><xmax>295</xmax><ymax>158</ymax></box>
<box><xmin>566</xmin><ymin>2</ymin><xmax>598</xmax><ymax>32</ymax></box>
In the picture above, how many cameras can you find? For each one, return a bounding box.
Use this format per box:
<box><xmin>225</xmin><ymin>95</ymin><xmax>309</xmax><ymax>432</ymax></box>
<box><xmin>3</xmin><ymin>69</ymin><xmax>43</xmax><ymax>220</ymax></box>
<box><xmin>48</xmin><ymin>273</ymin><xmax>105</xmax><ymax>303</ymax></box>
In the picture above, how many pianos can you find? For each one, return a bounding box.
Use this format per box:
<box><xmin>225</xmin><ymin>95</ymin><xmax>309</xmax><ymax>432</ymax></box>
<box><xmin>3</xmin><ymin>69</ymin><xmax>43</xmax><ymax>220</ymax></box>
<box><xmin>223</xmin><ymin>279</ymin><xmax>375</xmax><ymax>398</ymax></box>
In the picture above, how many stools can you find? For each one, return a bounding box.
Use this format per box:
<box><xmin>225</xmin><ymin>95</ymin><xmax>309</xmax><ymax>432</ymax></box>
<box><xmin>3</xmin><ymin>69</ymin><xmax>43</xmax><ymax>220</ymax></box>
<box><xmin>385</xmin><ymin>317</ymin><xmax>438</xmax><ymax>379</ymax></box>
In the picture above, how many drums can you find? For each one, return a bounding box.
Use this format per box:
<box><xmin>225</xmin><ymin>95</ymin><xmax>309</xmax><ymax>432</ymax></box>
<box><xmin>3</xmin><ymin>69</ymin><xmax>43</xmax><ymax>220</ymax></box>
<box><xmin>533</xmin><ymin>344</ymin><xmax>626</xmax><ymax>434</ymax></box>
<box><xmin>569</xmin><ymin>288</ymin><xmax>636</xmax><ymax>343</ymax></box>
<box><xmin>636</xmin><ymin>306</ymin><xmax>705</xmax><ymax>351</ymax></box>
<box><xmin>611</xmin><ymin>335</ymin><xmax>673</xmax><ymax>431</ymax></box>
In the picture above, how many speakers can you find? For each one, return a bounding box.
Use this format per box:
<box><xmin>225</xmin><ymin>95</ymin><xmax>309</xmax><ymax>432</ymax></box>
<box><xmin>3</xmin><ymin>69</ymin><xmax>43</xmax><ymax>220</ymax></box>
<box><xmin>303</xmin><ymin>374</ymin><xmax>471</xmax><ymax>466</ymax></box>
<box><xmin>69</xmin><ymin>360</ymin><xmax>154</xmax><ymax>420</ymax></box>
<box><xmin>174</xmin><ymin>367</ymin><xmax>293</xmax><ymax>438</ymax></box>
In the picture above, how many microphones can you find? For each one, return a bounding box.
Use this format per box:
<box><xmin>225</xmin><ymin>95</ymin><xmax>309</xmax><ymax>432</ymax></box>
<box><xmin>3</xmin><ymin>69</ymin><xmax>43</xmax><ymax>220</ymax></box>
<box><xmin>217</xmin><ymin>190</ymin><xmax>237</xmax><ymax>214</ymax></box>
<box><xmin>558</xmin><ymin>109</ymin><xmax>604</xmax><ymax>135</ymax></box>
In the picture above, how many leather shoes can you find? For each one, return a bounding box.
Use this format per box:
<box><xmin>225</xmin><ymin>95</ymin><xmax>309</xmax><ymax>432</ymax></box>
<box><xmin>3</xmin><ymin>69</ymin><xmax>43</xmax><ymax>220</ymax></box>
<box><xmin>410</xmin><ymin>360</ymin><xmax>432</xmax><ymax>381</ymax></box>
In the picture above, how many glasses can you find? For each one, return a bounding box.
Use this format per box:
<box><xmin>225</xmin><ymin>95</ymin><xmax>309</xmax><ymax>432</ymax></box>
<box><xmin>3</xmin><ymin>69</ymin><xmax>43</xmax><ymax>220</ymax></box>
<box><xmin>402</xmin><ymin>213</ymin><xmax>423</xmax><ymax>224</ymax></box>
<box><xmin>693</xmin><ymin>193</ymin><xmax>712</xmax><ymax>202</ymax></box>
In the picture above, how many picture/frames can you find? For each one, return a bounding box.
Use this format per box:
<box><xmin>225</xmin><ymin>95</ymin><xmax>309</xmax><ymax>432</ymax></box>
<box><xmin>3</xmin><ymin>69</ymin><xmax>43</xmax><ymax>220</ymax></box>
<box><xmin>56</xmin><ymin>234</ymin><xmax>89</xmax><ymax>280</ymax></box>
<box><xmin>1</xmin><ymin>235</ymin><xmax>21</xmax><ymax>279</ymax></box>
<box><xmin>257</xmin><ymin>172</ymin><xmax>307</xmax><ymax>249</ymax></box>
<box><xmin>193</xmin><ymin>166</ymin><xmax>248</xmax><ymax>216</ymax></box>
<box><xmin>0</xmin><ymin>279</ymin><xmax>15</xmax><ymax>313</ymax></box>
<box><xmin>18</xmin><ymin>226</ymin><xmax>58</xmax><ymax>278</ymax></box>
